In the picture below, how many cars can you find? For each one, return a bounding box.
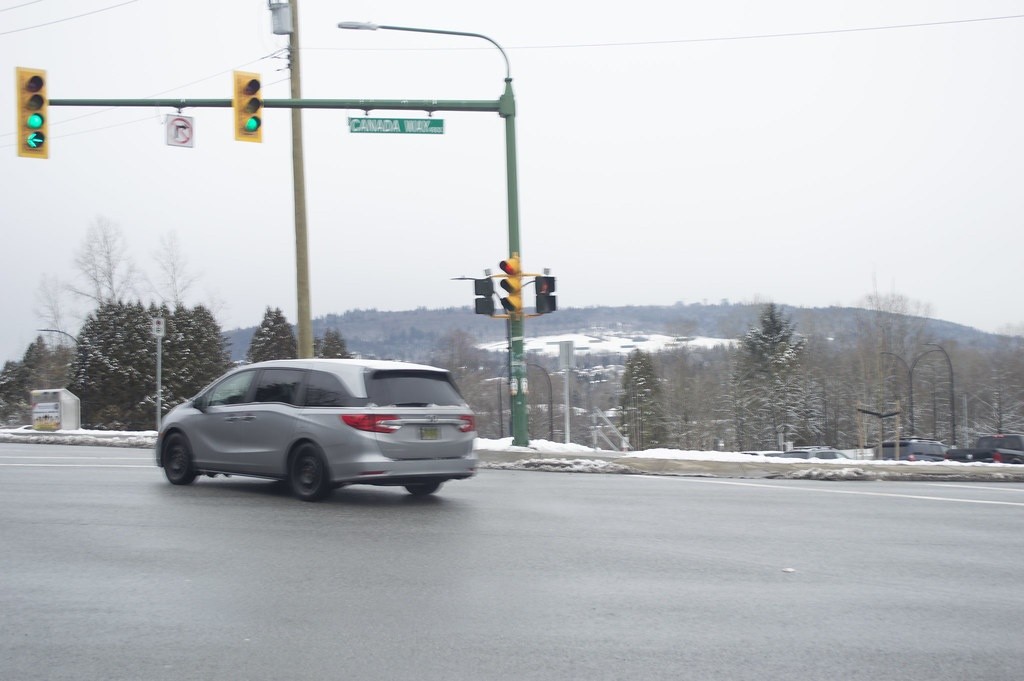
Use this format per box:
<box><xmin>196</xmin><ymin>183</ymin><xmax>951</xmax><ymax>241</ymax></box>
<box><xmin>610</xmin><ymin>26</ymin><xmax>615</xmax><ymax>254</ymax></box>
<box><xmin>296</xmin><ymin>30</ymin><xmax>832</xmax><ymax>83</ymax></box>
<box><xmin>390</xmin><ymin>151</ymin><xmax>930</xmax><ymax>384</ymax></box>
<box><xmin>156</xmin><ymin>358</ymin><xmax>477</xmax><ymax>504</ymax></box>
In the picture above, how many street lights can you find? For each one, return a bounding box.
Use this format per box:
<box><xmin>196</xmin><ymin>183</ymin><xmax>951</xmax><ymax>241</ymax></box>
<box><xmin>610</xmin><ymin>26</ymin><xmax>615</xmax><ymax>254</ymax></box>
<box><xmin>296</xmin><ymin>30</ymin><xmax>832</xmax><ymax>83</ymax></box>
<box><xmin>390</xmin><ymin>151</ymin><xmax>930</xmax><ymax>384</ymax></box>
<box><xmin>880</xmin><ymin>349</ymin><xmax>942</xmax><ymax>437</ymax></box>
<box><xmin>922</xmin><ymin>342</ymin><xmax>955</xmax><ymax>445</ymax></box>
<box><xmin>336</xmin><ymin>22</ymin><xmax>531</xmax><ymax>446</ymax></box>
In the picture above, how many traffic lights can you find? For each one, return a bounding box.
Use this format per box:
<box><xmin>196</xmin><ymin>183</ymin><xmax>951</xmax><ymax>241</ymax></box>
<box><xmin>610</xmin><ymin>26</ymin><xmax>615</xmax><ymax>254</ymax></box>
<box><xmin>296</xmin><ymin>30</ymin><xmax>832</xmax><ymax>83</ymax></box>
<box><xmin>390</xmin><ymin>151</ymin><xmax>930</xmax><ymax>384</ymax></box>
<box><xmin>234</xmin><ymin>69</ymin><xmax>264</xmax><ymax>144</ymax></box>
<box><xmin>534</xmin><ymin>276</ymin><xmax>559</xmax><ymax>321</ymax></box>
<box><xmin>498</xmin><ymin>257</ymin><xmax>525</xmax><ymax>316</ymax></box>
<box><xmin>470</xmin><ymin>277</ymin><xmax>495</xmax><ymax>315</ymax></box>
<box><xmin>14</xmin><ymin>63</ymin><xmax>48</xmax><ymax>156</ymax></box>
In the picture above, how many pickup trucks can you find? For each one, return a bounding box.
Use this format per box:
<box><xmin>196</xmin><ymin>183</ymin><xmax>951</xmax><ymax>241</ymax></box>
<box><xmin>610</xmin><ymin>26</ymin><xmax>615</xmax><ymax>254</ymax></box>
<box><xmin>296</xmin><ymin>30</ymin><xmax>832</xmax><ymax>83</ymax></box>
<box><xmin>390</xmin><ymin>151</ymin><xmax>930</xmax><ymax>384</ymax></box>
<box><xmin>944</xmin><ymin>433</ymin><xmax>1024</xmax><ymax>464</ymax></box>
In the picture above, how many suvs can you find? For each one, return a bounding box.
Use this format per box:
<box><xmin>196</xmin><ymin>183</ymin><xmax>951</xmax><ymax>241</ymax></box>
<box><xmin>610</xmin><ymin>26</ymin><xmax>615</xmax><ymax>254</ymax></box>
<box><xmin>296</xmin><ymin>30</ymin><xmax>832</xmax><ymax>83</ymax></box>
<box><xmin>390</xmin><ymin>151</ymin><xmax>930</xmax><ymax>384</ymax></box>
<box><xmin>736</xmin><ymin>436</ymin><xmax>949</xmax><ymax>462</ymax></box>
